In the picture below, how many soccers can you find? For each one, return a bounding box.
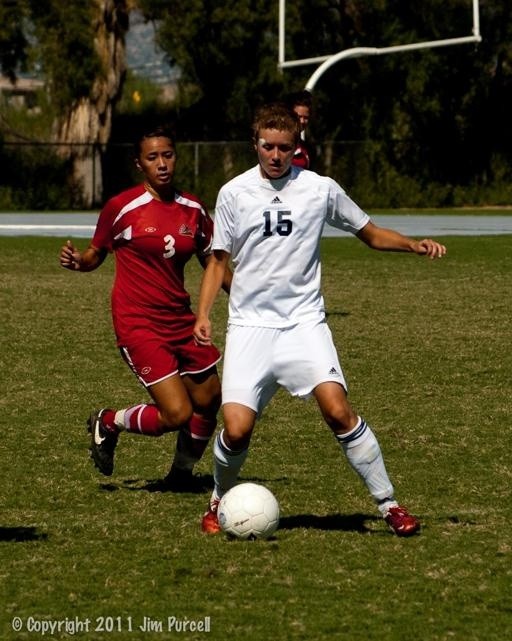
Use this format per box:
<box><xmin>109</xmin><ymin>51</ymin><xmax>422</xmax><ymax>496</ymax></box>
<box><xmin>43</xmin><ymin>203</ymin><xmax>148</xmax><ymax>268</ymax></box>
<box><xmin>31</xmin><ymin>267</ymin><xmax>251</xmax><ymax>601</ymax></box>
<box><xmin>218</xmin><ymin>483</ymin><xmax>279</xmax><ymax>541</ymax></box>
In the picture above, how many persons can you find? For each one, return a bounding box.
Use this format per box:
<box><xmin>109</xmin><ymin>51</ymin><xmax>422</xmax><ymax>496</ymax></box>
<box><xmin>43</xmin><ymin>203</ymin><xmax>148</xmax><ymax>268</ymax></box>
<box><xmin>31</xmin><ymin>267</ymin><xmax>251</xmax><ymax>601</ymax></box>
<box><xmin>192</xmin><ymin>105</ymin><xmax>447</xmax><ymax>536</ymax></box>
<box><xmin>58</xmin><ymin>127</ymin><xmax>233</xmax><ymax>489</ymax></box>
<box><xmin>287</xmin><ymin>88</ymin><xmax>317</xmax><ymax>171</ymax></box>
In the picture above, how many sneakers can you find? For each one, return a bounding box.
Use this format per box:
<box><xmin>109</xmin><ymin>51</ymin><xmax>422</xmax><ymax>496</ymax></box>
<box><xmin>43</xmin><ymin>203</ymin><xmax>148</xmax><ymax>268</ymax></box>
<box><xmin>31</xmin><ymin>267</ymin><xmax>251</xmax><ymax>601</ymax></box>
<box><xmin>86</xmin><ymin>409</ymin><xmax>119</xmax><ymax>475</ymax></box>
<box><xmin>203</xmin><ymin>491</ymin><xmax>221</xmax><ymax>533</ymax></box>
<box><xmin>385</xmin><ymin>507</ymin><xmax>420</xmax><ymax>535</ymax></box>
<box><xmin>166</xmin><ymin>467</ymin><xmax>207</xmax><ymax>494</ymax></box>
